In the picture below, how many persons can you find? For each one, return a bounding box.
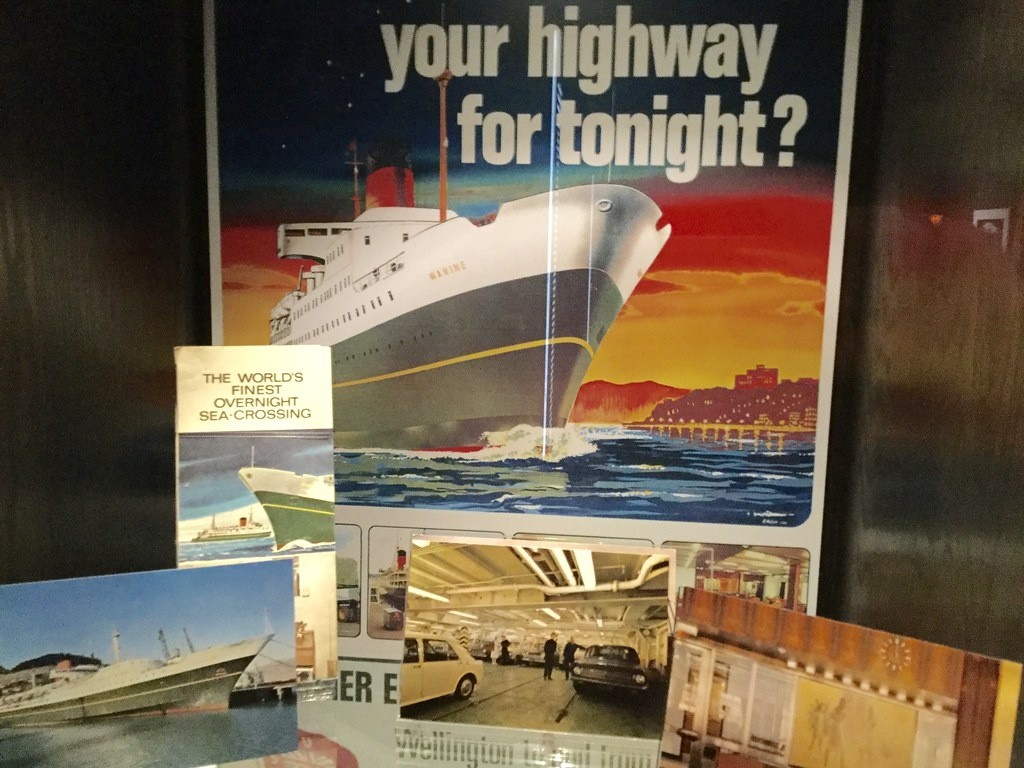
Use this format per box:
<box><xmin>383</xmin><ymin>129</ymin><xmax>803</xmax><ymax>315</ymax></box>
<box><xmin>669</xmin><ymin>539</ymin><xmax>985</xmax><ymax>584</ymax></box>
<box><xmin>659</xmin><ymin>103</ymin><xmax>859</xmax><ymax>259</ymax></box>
<box><xmin>514</xmin><ymin>652</ymin><xmax>525</xmax><ymax>665</ymax></box>
<box><xmin>562</xmin><ymin>636</ymin><xmax>588</xmax><ymax>681</ymax></box>
<box><xmin>544</xmin><ymin>631</ymin><xmax>558</xmax><ymax>681</ymax></box>
<box><xmin>500</xmin><ymin>634</ymin><xmax>512</xmax><ymax>666</ymax></box>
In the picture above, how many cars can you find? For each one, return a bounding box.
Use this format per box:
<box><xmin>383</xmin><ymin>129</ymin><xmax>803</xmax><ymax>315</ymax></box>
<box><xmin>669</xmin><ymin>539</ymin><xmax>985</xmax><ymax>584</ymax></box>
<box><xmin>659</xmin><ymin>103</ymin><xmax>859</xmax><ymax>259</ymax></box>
<box><xmin>570</xmin><ymin>645</ymin><xmax>649</xmax><ymax>701</ymax></box>
<box><xmin>400</xmin><ymin>632</ymin><xmax>484</xmax><ymax>708</ymax></box>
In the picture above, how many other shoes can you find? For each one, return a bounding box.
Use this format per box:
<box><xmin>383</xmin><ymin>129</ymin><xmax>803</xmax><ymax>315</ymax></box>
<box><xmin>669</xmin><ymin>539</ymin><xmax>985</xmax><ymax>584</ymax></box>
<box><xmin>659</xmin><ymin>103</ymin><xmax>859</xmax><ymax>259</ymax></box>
<box><xmin>565</xmin><ymin>677</ymin><xmax>568</xmax><ymax>680</ymax></box>
<box><xmin>548</xmin><ymin>676</ymin><xmax>552</xmax><ymax>680</ymax></box>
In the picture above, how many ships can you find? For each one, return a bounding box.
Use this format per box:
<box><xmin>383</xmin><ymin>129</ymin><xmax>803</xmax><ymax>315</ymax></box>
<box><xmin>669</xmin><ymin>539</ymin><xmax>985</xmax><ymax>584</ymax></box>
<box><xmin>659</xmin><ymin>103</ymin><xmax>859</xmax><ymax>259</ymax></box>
<box><xmin>262</xmin><ymin>73</ymin><xmax>673</xmax><ymax>457</ymax></box>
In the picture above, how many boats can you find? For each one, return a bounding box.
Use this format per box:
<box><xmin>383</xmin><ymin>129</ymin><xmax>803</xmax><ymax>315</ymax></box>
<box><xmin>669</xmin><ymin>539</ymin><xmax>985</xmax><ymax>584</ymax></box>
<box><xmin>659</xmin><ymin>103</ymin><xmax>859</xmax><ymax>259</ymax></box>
<box><xmin>192</xmin><ymin>508</ymin><xmax>272</xmax><ymax>542</ymax></box>
<box><xmin>0</xmin><ymin>626</ymin><xmax>274</xmax><ymax>728</ymax></box>
<box><xmin>238</xmin><ymin>445</ymin><xmax>335</xmax><ymax>551</ymax></box>
